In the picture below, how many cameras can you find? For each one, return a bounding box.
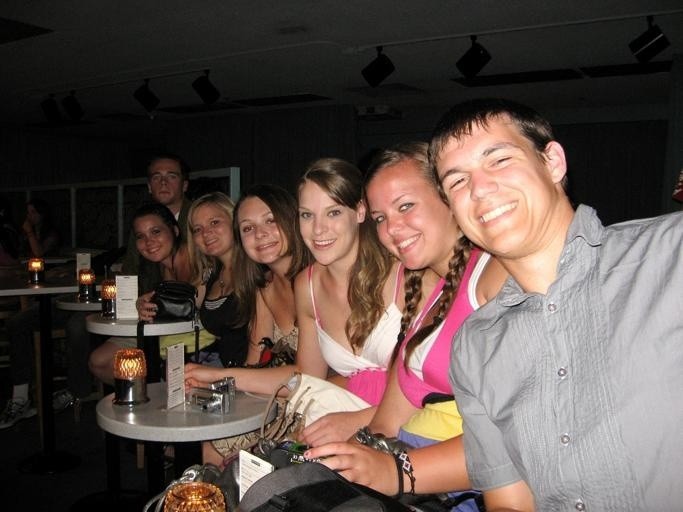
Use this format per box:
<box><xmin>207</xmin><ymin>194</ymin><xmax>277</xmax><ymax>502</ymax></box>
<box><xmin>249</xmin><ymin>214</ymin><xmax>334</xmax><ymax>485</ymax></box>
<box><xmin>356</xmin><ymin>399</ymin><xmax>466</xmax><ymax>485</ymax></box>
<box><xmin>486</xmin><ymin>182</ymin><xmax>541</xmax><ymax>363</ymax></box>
<box><xmin>209</xmin><ymin>377</ymin><xmax>237</xmax><ymax>400</ymax></box>
<box><xmin>190</xmin><ymin>389</ymin><xmax>229</xmax><ymax>414</ymax></box>
<box><xmin>355</xmin><ymin>426</ymin><xmax>405</xmax><ymax>454</ymax></box>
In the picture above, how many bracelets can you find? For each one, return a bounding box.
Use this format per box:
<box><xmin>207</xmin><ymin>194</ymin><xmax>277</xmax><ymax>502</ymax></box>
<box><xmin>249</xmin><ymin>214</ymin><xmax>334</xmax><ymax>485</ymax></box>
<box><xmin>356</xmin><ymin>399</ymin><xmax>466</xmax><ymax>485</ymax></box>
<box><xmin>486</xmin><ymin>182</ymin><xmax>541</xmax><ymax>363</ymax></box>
<box><xmin>26</xmin><ymin>230</ymin><xmax>32</xmax><ymax>234</ymax></box>
<box><xmin>26</xmin><ymin>235</ymin><xmax>33</xmax><ymax>238</ymax></box>
<box><xmin>392</xmin><ymin>449</ymin><xmax>405</xmax><ymax>499</ymax></box>
<box><xmin>396</xmin><ymin>449</ymin><xmax>417</xmax><ymax>495</ymax></box>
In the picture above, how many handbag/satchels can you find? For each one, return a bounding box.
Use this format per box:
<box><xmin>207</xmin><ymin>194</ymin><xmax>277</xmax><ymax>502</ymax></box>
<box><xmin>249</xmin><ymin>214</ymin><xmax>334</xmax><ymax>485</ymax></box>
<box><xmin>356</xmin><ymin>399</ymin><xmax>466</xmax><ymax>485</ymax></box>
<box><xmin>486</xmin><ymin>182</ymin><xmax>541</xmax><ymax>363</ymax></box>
<box><xmin>152</xmin><ymin>280</ymin><xmax>198</xmax><ymax>321</ymax></box>
<box><xmin>262</xmin><ymin>369</ymin><xmax>372</xmax><ymax>441</ymax></box>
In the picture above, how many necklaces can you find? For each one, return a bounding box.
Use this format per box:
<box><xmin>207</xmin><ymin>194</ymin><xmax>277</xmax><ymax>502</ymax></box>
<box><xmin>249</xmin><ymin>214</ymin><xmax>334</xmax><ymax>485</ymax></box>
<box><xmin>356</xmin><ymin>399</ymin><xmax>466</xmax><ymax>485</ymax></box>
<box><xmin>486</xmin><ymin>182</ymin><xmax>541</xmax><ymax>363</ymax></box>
<box><xmin>215</xmin><ymin>267</ymin><xmax>232</xmax><ymax>297</ymax></box>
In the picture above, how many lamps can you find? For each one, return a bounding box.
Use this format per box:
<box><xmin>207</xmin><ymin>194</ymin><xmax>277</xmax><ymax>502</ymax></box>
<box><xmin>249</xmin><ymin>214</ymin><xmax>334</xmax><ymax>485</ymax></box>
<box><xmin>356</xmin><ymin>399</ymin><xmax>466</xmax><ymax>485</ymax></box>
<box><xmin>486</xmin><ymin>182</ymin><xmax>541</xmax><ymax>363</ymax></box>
<box><xmin>39</xmin><ymin>70</ymin><xmax>222</xmax><ymax>122</ymax></box>
<box><xmin>360</xmin><ymin>13</ymin><xmax>672</xmax><ymax>88</ymax></box>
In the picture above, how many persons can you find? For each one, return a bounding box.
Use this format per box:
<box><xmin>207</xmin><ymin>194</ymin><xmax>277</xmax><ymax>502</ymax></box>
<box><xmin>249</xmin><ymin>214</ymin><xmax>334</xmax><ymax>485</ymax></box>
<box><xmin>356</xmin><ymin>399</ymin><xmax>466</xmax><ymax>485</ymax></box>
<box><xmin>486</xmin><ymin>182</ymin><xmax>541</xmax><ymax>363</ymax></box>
<box><xmin>126</xmin><ymin>196</ymin><xmax>223</xmax><ymax>379</ymax></box>
<box><xmin>14</xmin><ymin>190</ymin><xmax>60</xmax><ymax>311</ymax></box>
<box><xmin>87</xmin><ymin>152</ymin><xmax>195</xmax><ymax>392</ymax></box>
<box><xmin>192</xmin><ymin>182</ymin><xmax>351</xmax><ymax>474</ymax></box>
<box><xmin>182</xmin><ymin>153</ymin><xmax>406</xmax><ymax>510</ymax></box>
<box><xmin>135</xmin><ymin>189</ymin><xmax>266</xmax><ymax>367</ymax></box>
<box><xmin>426</xmin><ymin>99</ymin><xmax>683</xmax><ymax>512</ymax></box>
<box><xmin>232</xmin><ymin>138</ymin><xmax>509</xmax><ymax>511</ymax></box>
<box><xmin>0</xmin><ymin>297</ymin><xmax>76</xmax><ymax>430</ymax></box>
